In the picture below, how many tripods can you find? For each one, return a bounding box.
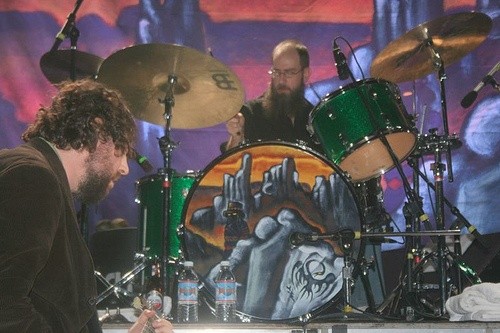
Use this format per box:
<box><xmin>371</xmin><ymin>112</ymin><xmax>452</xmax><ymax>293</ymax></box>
<box><xmin>95</xmin><ymin>75</ymin><xmax>185</xmax><ymax>306</ymax></box>
<box><xmin>374</xmin><ymin>42</ymin><xmax>487</xmax><ymax>323</ymax></box>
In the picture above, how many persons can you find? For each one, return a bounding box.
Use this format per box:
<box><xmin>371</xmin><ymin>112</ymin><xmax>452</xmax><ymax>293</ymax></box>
<box><xmin>1</xmin><ymin>80</ymin><xmax>175</xmax><ymax>333</ymax></box>
<box><xmin>218</xmin><ymin>40</ymin><xmax>319</xmax><ymax>153</ymax></box>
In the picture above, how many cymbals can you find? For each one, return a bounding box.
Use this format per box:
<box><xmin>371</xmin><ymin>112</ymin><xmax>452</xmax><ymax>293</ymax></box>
<box><xmin>368</xmin><ymin>11</ymin><xmax>493</xmax><ymax>82</ymax></box>
<box><xmin>419</xmin><ymin>129</ymin><xmax>463</xmax><ymax>156</ymax></box>
<box><xmin>96</xmin><ymin>40</ymin><xmax>246</xmax><ymax>131</ymax></box>
<box><xmin>38</xmin><ymin>44</ymin><xmax>105</xmax><ymax>90</ymax></box>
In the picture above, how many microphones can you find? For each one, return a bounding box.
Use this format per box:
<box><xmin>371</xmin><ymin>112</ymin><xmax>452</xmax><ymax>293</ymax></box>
<box><xmin>334</xmin><ymin>40</ymin><xmax>349</xmax><ymax>81</ymax></box>
<box><xmin>460</xmin><ymin>61</ymin><xmax>500</xmax><ymax>108</ymax></box>
<box><xmin>290</xmin><ymin>232</ymin><xmax>305</xmax><ymax>247</ymax></box>
<box><xmin>132</xmin><ymin>151</ymin><xmax>152</xmax><ymax>171</ymax></box>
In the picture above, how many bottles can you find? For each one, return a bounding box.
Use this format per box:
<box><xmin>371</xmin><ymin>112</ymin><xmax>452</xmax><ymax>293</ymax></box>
<box><xmin>176</xmin><ymin>261</ymin><xmax>199</xmax><ymax>324</ymax></box>
<box><xmin>215</xmin><ymin>261</ymin><xmax>237</xmax><ymax>323</ymax></box>
<box><xmin>145</xmin><ymin>291</ymin><xmax>162</xmax><ymax>333</ymax></box>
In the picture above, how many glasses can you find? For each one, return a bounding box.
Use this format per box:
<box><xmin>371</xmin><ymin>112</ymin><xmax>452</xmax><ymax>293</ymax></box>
<box><xmin>266</xmin><ymin>65</ymin><xmax>305</xmax><ymax>78</ymax></box>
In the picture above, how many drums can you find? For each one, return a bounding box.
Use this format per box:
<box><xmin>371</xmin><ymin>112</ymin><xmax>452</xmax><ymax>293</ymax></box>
<box><xmin>177</xmin><ymin>138</ymin><xmax>365</xmax><ymax>325</ymax></box>
<box><xmin>130</xmin><ymin>164</ymin><xmax>204</xmax><ymax>293</ymax></box>
<box><xmin>307</xmin><ymin>76</ymin><xmax>421</xmax><ymax>185</ymax></box>
